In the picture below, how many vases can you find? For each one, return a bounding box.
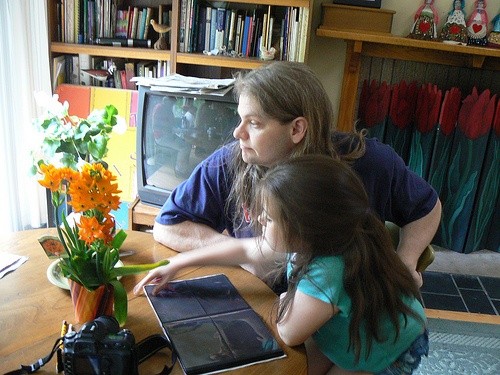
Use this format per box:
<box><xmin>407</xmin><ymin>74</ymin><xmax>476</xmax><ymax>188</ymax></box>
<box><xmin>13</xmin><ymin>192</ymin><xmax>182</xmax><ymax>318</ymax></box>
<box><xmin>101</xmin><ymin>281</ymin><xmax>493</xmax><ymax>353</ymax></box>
<box><xmin>69</xmin><ymin>278</ymin><xmax>113</xmax><ymax>326</ymax></box>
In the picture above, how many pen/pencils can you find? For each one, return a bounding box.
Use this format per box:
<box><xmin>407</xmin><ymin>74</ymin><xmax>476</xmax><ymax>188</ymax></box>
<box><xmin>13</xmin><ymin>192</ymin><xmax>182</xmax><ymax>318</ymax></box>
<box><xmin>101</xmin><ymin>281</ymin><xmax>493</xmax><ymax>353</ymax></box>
<box><xmin>68</xmin><ymin>324</ymin><xmax>72</xmax><ymax>332</ymax></box>
<box><xmin>60</xmin><ymin>320</ymin><xmax>66</xmax><ymax>349</ymax></box>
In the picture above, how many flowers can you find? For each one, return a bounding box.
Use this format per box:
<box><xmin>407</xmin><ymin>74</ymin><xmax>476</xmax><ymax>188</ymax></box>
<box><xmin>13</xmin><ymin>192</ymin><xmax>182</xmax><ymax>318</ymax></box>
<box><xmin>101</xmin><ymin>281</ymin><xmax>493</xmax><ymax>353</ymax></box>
<box><xmin>28</xmin><ymin>94</ymin><xmax>169</xmax><ymax>327</ymax></box>
<box><xmin>362</xmin><ymin>79</ymin><xmax>500</xmax><ymax>254</ymax></box>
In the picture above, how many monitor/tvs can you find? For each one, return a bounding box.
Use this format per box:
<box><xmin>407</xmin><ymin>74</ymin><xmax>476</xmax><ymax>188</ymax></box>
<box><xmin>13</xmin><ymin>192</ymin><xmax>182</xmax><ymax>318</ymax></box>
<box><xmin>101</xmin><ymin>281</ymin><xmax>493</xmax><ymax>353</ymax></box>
<box><xmin>139</xmin><ymin>86</ymin><xmax>243</xmax><ymax>205</ymax></box>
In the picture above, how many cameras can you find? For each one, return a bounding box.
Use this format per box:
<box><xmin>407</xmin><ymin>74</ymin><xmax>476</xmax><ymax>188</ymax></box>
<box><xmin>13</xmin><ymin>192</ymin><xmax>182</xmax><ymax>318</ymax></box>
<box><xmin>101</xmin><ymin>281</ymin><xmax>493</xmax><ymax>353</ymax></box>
<box><xmin>65</xmin><ymin>316</ymin><xmax>139</xmax><ymax>375</ymax></box>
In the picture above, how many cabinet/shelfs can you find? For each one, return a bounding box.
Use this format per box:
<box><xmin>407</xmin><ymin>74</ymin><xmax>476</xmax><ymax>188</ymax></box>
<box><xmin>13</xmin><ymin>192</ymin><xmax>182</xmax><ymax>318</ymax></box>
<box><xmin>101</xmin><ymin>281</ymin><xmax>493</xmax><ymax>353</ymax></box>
<box><xmin>47</xmin><ymin>1</ymin><xmax>314</xmax><ymax>97</ymax></box>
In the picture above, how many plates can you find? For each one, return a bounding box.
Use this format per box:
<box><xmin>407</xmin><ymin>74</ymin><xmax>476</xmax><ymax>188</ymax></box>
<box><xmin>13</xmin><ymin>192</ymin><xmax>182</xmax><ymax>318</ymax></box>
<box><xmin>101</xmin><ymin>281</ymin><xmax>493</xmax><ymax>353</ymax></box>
<box><xmin>47</xmin><ymin>258</ymin><xmax>124</xmax><ymax>290</ymax></box>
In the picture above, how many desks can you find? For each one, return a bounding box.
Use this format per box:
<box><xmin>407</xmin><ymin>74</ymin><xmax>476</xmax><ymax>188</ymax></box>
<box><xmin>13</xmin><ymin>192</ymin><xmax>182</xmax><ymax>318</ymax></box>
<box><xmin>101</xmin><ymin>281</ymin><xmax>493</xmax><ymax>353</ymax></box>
<box><xmin>0</xmin><ymin>228</ymin><xmax>309</xmax><ymax>374</ymax></box>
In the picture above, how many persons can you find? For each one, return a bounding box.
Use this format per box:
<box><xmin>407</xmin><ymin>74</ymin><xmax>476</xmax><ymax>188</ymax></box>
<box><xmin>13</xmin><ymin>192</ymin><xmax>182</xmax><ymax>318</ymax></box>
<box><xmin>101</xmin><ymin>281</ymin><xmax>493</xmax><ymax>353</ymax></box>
<box><xmin>151</xmin><ymin>96</ymin><xmax>197</xmax><ymax>150</ymax></box>
<box><xmin>410</xmin><ymin>0</ymin><xmax>437</xmax><ymax>38</ymax></box>
<box><xmin>465</xmin><ymin>0</ymin><xmax>489</xmax><ymax>45</ymax></box>
<box><xmin>440</xmin><ymin>0</ymin><xmax>468</xmax><ymax>43</ymax></box>
<box><xmin>133</xmin><ymin>153</ymin><xmax>429</xmax><ymax>375</ymax></box>
<box><xmin>487</xmin><ymin>13</ymin><xmax>500</xmax><ymax>45</ymax></box>
<box><xmin>153</xmin><ymin>61</ymin><xmax>441</xmax><ymax>296</ymax></box>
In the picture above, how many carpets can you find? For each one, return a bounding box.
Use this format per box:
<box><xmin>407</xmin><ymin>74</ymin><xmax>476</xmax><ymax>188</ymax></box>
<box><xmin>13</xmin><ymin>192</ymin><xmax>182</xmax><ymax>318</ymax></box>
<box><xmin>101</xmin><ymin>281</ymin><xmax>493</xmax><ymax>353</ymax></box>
<box><xmin>413</xmin><ymin>317</ymin><xmax>499</xmax><ymax>375</ymax></box>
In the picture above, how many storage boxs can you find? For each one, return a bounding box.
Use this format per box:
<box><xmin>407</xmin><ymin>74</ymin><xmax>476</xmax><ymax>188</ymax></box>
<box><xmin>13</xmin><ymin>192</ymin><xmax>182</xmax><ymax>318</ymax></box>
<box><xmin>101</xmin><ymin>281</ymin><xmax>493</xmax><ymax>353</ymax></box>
<box><xmin>321</xmin><ymin>3</ymin><xmax>396</xmax><ymax>33</ymax></box>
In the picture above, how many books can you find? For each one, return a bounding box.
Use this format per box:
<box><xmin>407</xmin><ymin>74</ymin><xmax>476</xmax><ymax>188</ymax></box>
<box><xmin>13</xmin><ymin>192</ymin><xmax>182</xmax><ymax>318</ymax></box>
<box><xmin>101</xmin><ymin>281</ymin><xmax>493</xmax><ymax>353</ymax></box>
<box><xmin>52</xmin><ymin>0</ymin><xmax>299</xmax><ymax>98</ymax></box>
